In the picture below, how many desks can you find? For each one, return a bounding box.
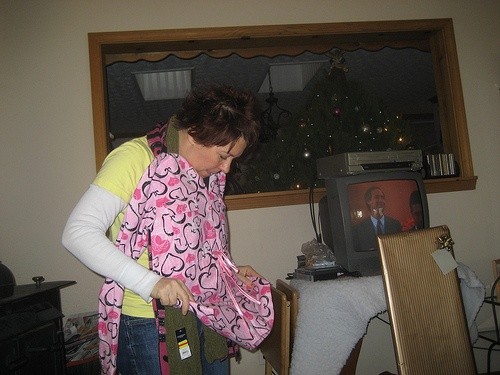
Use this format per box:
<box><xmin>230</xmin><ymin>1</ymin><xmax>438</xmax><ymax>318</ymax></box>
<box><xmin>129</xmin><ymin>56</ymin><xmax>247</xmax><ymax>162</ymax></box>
<box><xmin>259</xmin><ymin>278</ymin><xmax>297</xmax><ymax>375</ymax></box>
<box><xmin>288</xmin><ymin>262</ymin><xmax>485</xmax><ymax>375</ymax></box>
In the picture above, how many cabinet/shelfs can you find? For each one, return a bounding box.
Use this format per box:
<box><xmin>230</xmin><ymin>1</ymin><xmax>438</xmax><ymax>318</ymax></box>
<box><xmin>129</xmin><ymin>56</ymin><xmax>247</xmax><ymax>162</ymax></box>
<box><xmin>0</xmin><ymin>279</ymin><xmax>77</xmax><ymax>375</ymax></box>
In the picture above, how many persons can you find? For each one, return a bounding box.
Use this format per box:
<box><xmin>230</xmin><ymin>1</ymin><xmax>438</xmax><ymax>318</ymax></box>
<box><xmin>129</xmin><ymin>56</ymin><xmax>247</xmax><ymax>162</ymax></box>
<box><xmin>61</xmin><ymin>81</ymin><xmax>268</xmax><ymax>375</ymax></box>
<box><xmin>352</xmin><ymin>187</ymin><xmax>401</xmax><ymax>252</ymax></box>
<box><xmin>409</xmin><ymin>191</ymin><xmax>424</xmax><ymax>231</ymax></box>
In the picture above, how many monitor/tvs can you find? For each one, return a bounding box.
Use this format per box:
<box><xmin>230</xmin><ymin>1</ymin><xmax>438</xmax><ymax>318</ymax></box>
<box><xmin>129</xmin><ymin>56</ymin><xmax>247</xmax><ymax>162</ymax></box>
<box><xmin>318</xmin><ymin>172</ymin><xmax>430</xmax><ymax>278</ymax></box>
